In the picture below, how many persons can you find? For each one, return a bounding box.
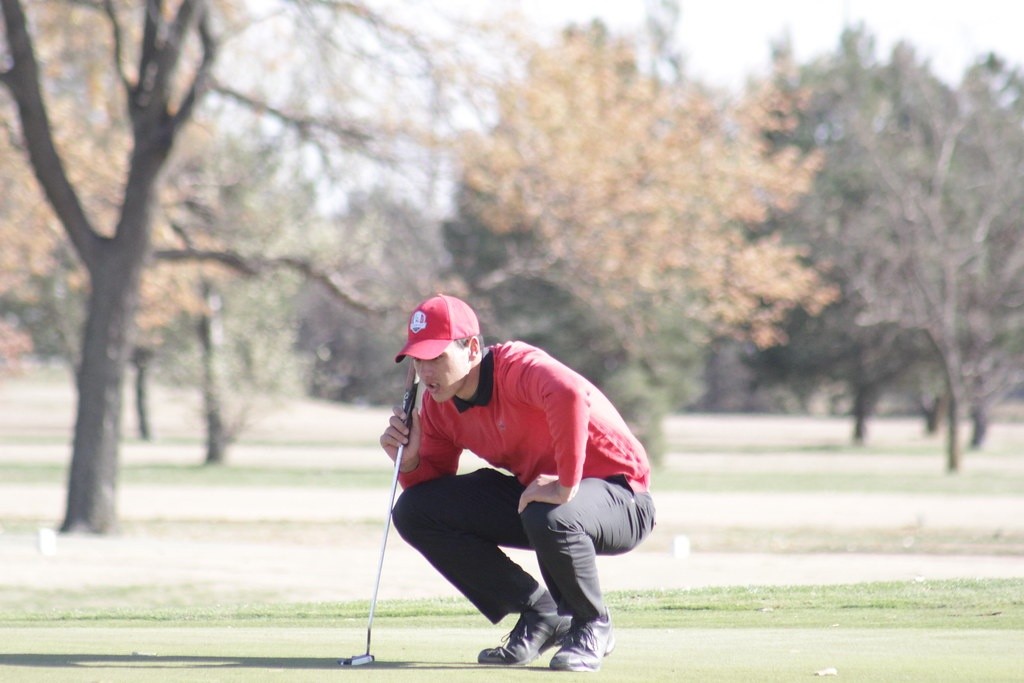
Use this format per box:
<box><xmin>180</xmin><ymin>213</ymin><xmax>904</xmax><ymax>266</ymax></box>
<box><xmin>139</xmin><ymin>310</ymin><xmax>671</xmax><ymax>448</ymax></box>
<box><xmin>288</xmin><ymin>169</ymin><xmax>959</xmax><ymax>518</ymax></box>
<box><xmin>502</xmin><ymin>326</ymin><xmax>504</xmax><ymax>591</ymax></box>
<box><xmin>378</xmin><ymin>293</ymin><xmax>655</xmax><ymax>672</ymax></box>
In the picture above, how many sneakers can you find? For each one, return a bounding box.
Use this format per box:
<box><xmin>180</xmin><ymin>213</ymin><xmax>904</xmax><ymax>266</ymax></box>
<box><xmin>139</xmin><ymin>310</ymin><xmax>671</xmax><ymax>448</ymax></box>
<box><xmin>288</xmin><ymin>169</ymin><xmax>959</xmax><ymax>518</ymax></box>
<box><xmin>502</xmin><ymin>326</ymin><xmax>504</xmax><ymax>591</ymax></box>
<box><xmin>549</xmin><ymin>610</ymin><xmax>614</xmax><ymax>672</ymax></box>
<box><xmin>475</xmin><ymin>601</ymin><xmax>572</xmax><ymax>666</ymax></box>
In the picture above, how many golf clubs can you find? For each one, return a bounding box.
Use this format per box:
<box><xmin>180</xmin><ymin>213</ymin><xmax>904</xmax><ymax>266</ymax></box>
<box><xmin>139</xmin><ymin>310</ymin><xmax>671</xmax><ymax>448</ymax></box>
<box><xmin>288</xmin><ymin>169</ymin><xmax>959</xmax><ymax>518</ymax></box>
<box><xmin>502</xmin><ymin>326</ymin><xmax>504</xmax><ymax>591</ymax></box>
<box><xmin>338</xmin><ymin>358</ymin><xmax>421</xmax><ymax>666</ymax></box>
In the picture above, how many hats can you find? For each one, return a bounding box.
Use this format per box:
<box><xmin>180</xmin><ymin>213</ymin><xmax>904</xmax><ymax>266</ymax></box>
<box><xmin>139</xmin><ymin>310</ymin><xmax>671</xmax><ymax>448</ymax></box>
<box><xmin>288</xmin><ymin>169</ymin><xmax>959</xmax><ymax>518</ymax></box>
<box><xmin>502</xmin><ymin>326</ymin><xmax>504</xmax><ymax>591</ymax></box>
<box><xmin>394</xmin><ymin>293</ymin><xmax>480</xmax><ymax>364</ymax></box>
